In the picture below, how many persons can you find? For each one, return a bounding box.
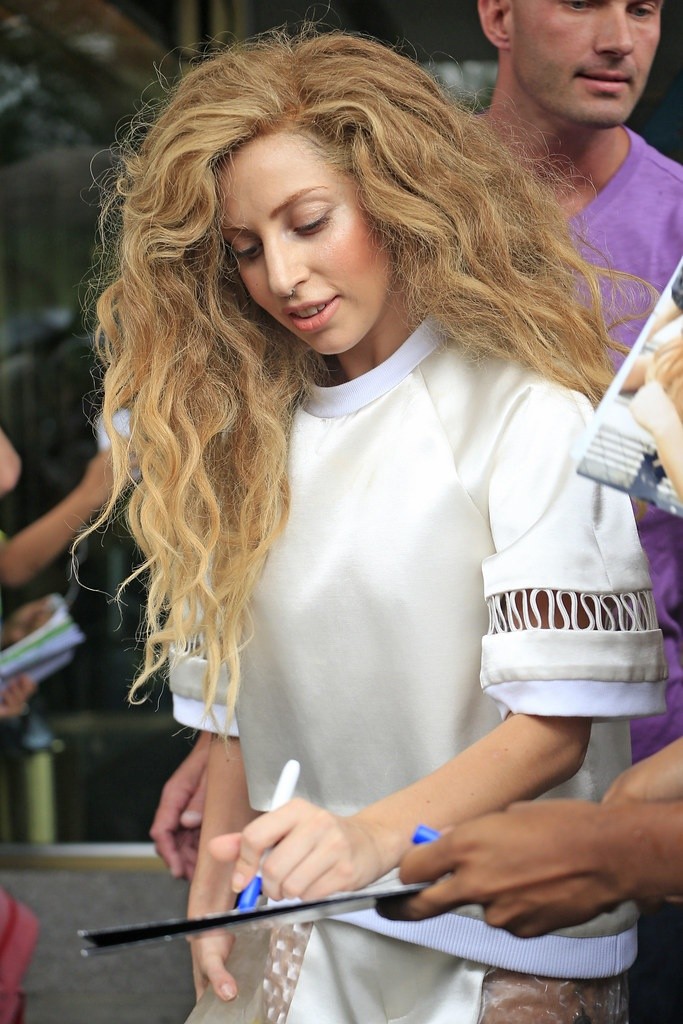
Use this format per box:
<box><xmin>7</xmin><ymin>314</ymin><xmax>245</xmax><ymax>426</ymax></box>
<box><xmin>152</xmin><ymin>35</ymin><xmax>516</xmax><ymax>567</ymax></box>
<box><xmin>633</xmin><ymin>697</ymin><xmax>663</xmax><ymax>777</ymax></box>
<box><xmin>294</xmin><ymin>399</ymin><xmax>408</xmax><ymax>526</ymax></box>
<box><xmin>0</xmin><ymin>0</ymin><xmax>683</xmax><ymax>1024</ymax></box>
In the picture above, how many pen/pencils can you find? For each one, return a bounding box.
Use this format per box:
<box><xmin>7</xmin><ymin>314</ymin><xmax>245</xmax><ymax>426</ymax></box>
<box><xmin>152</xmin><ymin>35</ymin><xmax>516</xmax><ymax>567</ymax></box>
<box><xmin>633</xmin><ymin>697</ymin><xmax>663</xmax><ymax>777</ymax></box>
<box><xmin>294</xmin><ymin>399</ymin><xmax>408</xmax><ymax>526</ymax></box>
<box><xmin>237</xmin><ymin>759</ymin><xmax>301</xmax><ymax>911</ymax></box>
<box><xmin>413</xmin><ymin>824</ymin><xmax>439</xmax><ymax>843</ymax></box>
